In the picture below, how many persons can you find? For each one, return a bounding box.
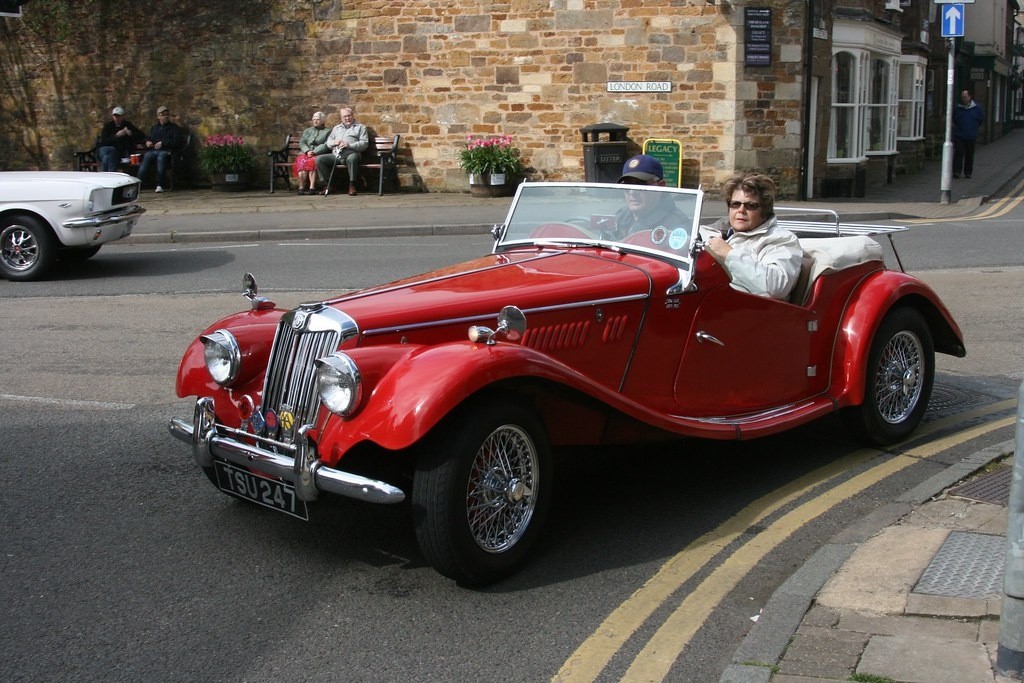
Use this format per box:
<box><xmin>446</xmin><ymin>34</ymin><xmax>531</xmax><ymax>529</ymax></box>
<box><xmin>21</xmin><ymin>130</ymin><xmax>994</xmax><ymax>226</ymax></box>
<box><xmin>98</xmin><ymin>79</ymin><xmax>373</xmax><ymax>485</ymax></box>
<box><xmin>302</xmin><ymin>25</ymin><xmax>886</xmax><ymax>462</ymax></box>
<box><xmin>315</xmin><ymin>108</ymin><xmax>368</xmax><ymax>196</ymax></box>
<box><xmin>96</xmin><ymin>107</ymin><xmax>145</xmax><ymax>172</ymax></box>
<box><xmin>296</xmin><ymin>112</ymin><xmax>332</xmax><ymax>194</ymax></box>
<box><xmin>952</xmin><ymin>89</ymin><xmax>982</xmax><ymax>179</ymax></box>
<box><xmin>136</xmin><ymin>106</ymin><xmax>180</xmax><ymax>193</ymax></box>
<box><xmin>696</xmin><ymin>174</ymin><xmax>803</xmax><ymax>300</ymax></box>
<box><xmin>596</xmin><ymin>154</ymin><xmax>694</xmax><ymax>242</ymax></box>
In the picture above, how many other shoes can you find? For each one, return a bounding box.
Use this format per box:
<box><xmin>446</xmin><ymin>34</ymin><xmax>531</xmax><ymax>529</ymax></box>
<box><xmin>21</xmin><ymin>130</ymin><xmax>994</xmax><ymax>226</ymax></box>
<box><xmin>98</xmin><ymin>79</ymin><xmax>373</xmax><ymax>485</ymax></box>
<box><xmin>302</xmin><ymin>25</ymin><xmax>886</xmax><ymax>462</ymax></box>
<box><xmin>349</xmin><ymin>185</ymin><xmax>357</xmax><ymax>195</ymax></box>
<box><xmin>298</xmin><ymin>189</ymin><xmax>304</xmax><ymax>194</ymax></box>
<box><xmin>965</xmin><ymin>174</ymin><xmax>971</xmax><ymax>178</ymax></box>
<box><xmin>155</xmin><ymin>186</ymin><xmax>164</xmax><ymax>193</ymax></box>
<box><xmin>318</xmin><ymin>186</ymin><xmax>326</xmax><ymax>194</ymax></box>
<box><xmin>953</xmin><ymin>174</ymin><xmax>960</xmax><ymax>178</ymax></box>
<box><xmin>309</xmin><ymin>189</ymin><xmax>316</xmax><ymax>195</ymax></box>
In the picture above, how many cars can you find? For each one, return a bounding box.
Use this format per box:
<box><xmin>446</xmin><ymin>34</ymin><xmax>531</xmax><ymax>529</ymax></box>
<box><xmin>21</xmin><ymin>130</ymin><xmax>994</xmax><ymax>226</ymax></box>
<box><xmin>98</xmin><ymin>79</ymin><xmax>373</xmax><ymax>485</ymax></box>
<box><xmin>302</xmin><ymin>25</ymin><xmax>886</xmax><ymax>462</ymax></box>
<box><xmin>168</xmin><ymin>176</ymin><xmax>969</xmax><ymax>591</ymax></box>
<box><xmin>0</xmin><ymin>170</ymin><xmax>147</xmax><ymax>283</ymax></box>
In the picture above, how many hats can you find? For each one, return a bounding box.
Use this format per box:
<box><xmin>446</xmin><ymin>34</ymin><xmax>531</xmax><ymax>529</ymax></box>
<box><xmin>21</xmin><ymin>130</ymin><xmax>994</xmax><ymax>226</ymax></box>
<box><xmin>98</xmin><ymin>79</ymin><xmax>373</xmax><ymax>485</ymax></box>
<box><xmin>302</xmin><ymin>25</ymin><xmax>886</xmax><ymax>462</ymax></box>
<box><xmin>157</xmin><ymin>106</ymin><xmax>170</xmax><ymax>113</ymax></box>
<box><xmin>617</xmin><ymin>155</ymin><xmax>663</xmax><ymax>183</ymax></box>
<box><xmin>113</xmin><ymin>107</ymin><xmax>125</xmax><ymax>116</ymax></box>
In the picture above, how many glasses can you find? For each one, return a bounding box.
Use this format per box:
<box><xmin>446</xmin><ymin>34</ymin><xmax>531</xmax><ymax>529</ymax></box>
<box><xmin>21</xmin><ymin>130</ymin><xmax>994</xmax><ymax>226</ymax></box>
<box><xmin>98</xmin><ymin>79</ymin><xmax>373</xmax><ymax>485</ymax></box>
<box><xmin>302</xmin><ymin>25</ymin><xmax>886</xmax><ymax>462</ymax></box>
<box><xmin>728</xmin><ymin>201</ymin><xmax>762</xmax><ymax>211</ymax></box>
<box><xmin>622</xmin><ymin>181</ymin><xmax>648</xmax><ymax>191</ymax></box>
<box><xmin>159</xmin><ymin>113</ymin><xmax>170</xmax><ymax>116</ymax></box>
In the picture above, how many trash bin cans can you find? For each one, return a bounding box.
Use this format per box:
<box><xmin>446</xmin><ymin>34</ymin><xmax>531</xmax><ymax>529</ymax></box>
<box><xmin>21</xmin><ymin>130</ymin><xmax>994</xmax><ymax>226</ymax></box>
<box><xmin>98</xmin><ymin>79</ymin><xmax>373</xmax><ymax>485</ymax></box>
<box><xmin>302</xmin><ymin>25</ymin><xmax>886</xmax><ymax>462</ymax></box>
<box><xmin>579</xmin><ymin>122</ymin><xmax>631</xmax><ymax>183</ymax></box>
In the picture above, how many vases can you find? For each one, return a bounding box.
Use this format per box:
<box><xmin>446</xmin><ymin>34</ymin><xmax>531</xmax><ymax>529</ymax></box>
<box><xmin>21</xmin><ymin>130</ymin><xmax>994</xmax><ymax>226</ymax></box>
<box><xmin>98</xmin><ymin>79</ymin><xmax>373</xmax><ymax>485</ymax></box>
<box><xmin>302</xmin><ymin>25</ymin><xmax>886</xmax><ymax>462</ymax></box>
<box><xmin>210</xmin><ymin>171</ymin><xmax>246</xmax><ymax>192</ymax></box>
<box><xmin>469</xmin><ymin>173</ymin><xmax>486</xmax><ymax>185</ymax></box>
<box><xmin>489</xmin><ymin>174</ymin><xmax>505</xmax><ymax>185</ymax></box>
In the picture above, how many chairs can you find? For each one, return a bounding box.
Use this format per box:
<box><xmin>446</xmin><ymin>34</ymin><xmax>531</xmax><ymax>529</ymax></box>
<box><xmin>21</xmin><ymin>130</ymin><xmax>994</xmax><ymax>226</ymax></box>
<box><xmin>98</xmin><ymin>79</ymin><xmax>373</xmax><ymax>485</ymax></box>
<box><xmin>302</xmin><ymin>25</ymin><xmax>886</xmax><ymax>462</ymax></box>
<box><xmin>785</xmin><ymin>255</ymin><xmax>814</xmax><ymax>309</ymax></box>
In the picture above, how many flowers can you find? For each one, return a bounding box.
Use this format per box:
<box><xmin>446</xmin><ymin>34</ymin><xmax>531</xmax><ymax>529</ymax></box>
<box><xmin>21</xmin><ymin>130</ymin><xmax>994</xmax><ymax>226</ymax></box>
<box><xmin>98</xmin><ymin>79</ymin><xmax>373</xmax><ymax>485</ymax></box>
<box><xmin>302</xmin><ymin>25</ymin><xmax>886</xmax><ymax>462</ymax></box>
<box><xmin>461</xmin><ymin>135</ymin><xmax>519</xmax><ymax>172</ymax></box>
<box><xmin>200</xmin><ymin>134</ymin><xmax>253</xmax><ymax>174</ymax></box>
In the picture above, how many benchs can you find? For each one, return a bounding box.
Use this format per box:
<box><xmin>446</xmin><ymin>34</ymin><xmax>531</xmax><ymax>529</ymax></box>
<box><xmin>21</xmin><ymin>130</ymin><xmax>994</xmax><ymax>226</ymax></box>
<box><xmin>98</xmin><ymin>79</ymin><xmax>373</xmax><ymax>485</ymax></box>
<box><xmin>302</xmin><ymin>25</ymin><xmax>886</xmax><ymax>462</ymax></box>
<box><xmin>267</xmin><ymin>133</ymin><xmax>399</xmax><ymax>195</ymax></box>
<box><xmin>73</xmin><ymin>134</ymin><xmax>193</xmax><ymax>191</ymax></box>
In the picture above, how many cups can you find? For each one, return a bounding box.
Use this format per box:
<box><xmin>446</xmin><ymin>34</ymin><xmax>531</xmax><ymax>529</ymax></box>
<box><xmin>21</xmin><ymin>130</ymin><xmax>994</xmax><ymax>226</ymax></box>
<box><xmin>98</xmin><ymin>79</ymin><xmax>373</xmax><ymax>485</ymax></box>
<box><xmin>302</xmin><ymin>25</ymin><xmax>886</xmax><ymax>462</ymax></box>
<box><xmin>130</xmin><ymin>154</ymin><xmax>141</xmax><ymax>164</ymax></box>
